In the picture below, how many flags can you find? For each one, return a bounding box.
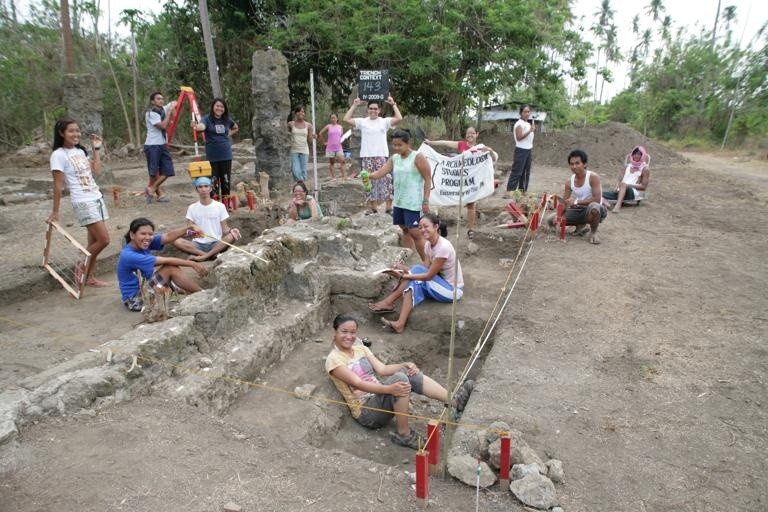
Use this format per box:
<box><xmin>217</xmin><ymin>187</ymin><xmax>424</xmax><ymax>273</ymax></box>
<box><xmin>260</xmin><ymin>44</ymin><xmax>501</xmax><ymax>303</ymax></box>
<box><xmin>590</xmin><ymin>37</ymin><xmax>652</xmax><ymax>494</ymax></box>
<box><xmin>417</xmin><ymin>141</ymin><xmax>496</xmax><ymax>207</ymax></box>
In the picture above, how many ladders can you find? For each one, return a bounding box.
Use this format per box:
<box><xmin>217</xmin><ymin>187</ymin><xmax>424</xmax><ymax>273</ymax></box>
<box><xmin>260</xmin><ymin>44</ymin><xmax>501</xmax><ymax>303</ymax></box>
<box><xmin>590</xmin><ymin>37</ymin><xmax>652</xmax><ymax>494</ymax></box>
<box><xmin>167</xmin><ymin>86</ymin><xmax>206</xmax><ymax>146</ymax></box>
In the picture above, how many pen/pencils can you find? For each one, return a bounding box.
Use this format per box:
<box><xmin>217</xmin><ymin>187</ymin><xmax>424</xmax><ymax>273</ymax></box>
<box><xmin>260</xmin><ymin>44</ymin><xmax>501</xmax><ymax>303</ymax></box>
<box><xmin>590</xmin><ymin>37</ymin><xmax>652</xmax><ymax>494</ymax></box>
<box><xmin>393</xmin><ymin>259</ymin><xmax>403</xmax><ymax>269</ymax></box>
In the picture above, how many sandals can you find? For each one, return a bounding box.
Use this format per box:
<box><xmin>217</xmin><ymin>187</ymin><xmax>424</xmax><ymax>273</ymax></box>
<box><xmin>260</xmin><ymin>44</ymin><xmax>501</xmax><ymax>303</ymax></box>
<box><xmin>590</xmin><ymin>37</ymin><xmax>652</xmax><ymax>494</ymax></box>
<box><xmin>145</xmin><ymin>187</ymin><xmax>170</xmax><ymax>204</ymax></box>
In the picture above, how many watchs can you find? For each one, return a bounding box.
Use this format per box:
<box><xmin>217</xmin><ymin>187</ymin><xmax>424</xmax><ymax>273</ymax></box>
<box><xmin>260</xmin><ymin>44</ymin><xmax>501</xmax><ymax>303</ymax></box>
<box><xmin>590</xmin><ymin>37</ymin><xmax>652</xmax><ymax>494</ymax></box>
<box><xmin>573</xmin><ymin>198</ymin><xmax>579</xmax><ymax>208</ymax></box>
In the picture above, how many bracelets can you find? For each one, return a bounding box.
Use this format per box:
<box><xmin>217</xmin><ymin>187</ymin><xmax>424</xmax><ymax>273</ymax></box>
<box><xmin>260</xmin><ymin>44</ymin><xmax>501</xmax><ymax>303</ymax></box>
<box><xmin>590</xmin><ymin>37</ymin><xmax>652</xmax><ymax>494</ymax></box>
<box><xmin>398</xmin><ymin>273</ymin><xmax>405</xmax><ymax>280</ymax></box>
<box><xmin>421</xmin><ymin>198</ymin><xmax>428</xmax><ymax>205</ymax></box>
<box><xmin>92</xmin><ymin>146</ymin><xmax>101</xmax><ymax>153</ymax></box>
<box><xmin>391</xmin><ymin>102</ymin><xmax>397</xmax><ymax>108</ymax></box>
<box><xmin>228</xmin><ymin>226</ymin><xmax>235</xmax><ymax>233</ymax></box>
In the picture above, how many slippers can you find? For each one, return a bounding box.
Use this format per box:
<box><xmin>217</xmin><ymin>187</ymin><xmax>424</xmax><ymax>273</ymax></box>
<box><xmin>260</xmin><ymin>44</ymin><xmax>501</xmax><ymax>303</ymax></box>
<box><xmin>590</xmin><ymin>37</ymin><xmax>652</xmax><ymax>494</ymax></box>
<box><xmin>368</xmin><ymin>303</ymin><xmax>394</xmax><ymax>334</ymax></box>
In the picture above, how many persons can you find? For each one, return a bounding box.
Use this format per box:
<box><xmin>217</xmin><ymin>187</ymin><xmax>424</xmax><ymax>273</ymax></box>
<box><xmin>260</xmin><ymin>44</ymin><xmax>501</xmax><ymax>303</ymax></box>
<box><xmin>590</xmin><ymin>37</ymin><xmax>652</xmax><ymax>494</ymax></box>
<box><xmin>365</xmin><ymin>128</ymin><xmax>432</xmax><ymax>265</ymax></box>
<box><xmin>318</xmin><ymin>112</ymin><xmax>349</xmax><ymax>181</ymax></box>
<box><xmin>343</xmin><ymin>93</ymin><xmax>402</xmax><ymax>215</ymax></box>
<box><xmin>168</xmin><ymin>175</ymin><xmax>242</xmax><ymax>263</ymax></box>
<box><xmin>543</xmin><ymin>150</ymin><xmax>607</xmax><ymax>245</ymax></box>
<box><xmin>44</xmin><ymin>117</ymin><xmax>109</xmax><ymax>288</ymax></box>
<box><xmin>288</xmin><ymin>104</ymin><xmax>318</xmax><ymax>183</ymax></box>
<box><xmin>506</xmin><ymin>104</ymin><xmax>537</xmax><ymax>196</ymax></box>
<box><xmin>422</xmin><ymin>123</ymin><xmax>498</xmax><ymax>238</ymax></box>
<box><xmin>283</xmin><ymin>182</ymin><xmax>325</xmax><ymax>226</ymax></box>
<box><xmin>117</xmin><ymin>217</ymin><xmax>209</xmax><ymax>310</ymax></box>
<box><xmin>366</xmin><ymin>212</ymin><xmax>465</xmax><ymax>335</ymax></box>
<box><xmin>601</xmin><ymin>144</ymin><xmax>650</xmax><ymax>213</ymax></box>
<box><xmin>143</xmin><ymin>91</ymin><xmax>178</xmax><ymax>205</ymax></box>
<box><xmin>322</xmin><ymin>312</ymin><xmax>476</xmax><ymax>453</ymax></box>
<box><xmin>189</xmin><ymin>96</ymin><xmax>239</xmax><ymax>210</ymax></box>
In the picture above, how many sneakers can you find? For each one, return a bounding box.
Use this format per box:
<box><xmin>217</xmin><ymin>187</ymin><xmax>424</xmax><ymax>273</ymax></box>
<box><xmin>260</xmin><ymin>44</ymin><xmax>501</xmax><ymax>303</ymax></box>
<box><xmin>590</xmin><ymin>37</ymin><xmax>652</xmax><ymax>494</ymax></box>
<box><xmin>390</xmin><ymin>427</ymin><xmax>427</xmax><ymax>450</ymax></box>
<box><xmin>454</xmin><ymin>379</ymin><xmax>473</xmax><ymax>413</ymax></box>
